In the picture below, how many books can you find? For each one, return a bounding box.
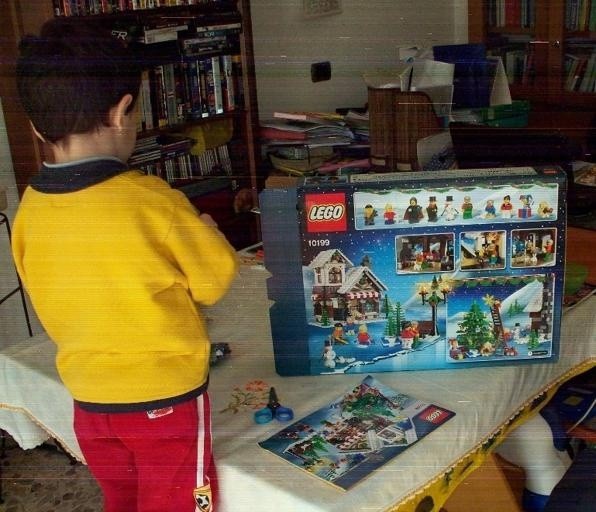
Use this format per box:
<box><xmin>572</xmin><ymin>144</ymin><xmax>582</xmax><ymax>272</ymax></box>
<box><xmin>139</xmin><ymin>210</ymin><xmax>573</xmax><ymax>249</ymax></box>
<box><xmin>260</xmin><ymin>107</ymin><xmax>370</xmax><ymax>176</ymax></box>
<box><xmin>54</xmin><ymin>1</ymin><xmax>236</xmax><ymax>185</ymax></box>
<box><xmin>483</xmin><ymin>0</ymin><xmax>595</xmax><ymax>94</ymax></box>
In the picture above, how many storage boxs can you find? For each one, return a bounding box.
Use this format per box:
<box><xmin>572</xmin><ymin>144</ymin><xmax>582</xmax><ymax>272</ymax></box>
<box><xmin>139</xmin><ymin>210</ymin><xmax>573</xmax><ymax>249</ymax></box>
<box><xmin>257</xmin><ymin>163</ymin><xmax>569</xmax><ymax>377</ymax></box>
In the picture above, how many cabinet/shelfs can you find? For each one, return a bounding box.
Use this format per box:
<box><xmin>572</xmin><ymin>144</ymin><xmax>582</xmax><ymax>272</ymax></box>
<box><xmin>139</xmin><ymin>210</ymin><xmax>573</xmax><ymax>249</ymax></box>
<box><xmin>0</xmin><ymin>0</ymin><xmax>263</xmax><ymax>252</ymax></box>
<box><xmin>466</xmin><ymin>0</ymin><xmax>596</xmax><ymax>109</ymax></box>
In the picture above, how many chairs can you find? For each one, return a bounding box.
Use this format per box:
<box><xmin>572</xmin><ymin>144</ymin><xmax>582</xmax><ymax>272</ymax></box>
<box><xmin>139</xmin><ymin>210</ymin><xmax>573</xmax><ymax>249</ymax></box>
<box><xmin>449</xmin><ymin>122</ymin><xmax>595</xmax><ymax>219</ymax></box>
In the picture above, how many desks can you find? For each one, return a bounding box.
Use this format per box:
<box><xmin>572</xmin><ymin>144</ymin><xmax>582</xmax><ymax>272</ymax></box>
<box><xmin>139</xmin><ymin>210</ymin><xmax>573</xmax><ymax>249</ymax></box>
<box><xmin>0</xmin><ymin>225</ymin><xmax>596</xmax><ymax>510</ymax></box>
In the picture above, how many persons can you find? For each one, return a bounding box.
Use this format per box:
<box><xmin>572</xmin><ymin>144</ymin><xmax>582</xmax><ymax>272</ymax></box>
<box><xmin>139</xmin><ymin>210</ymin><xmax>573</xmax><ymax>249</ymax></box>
<box><xmin>9</xmin><ymin>15</ymin><xmax>238</xmax><ymax>512</ymax></box>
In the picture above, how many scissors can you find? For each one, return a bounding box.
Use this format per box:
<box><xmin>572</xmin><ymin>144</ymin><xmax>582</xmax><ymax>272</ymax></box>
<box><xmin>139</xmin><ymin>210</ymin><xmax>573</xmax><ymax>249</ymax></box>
<box><xmin>254</xmin><ymin>386</ymin><xmax>293</xmax><ymax>424</ymax></box>
